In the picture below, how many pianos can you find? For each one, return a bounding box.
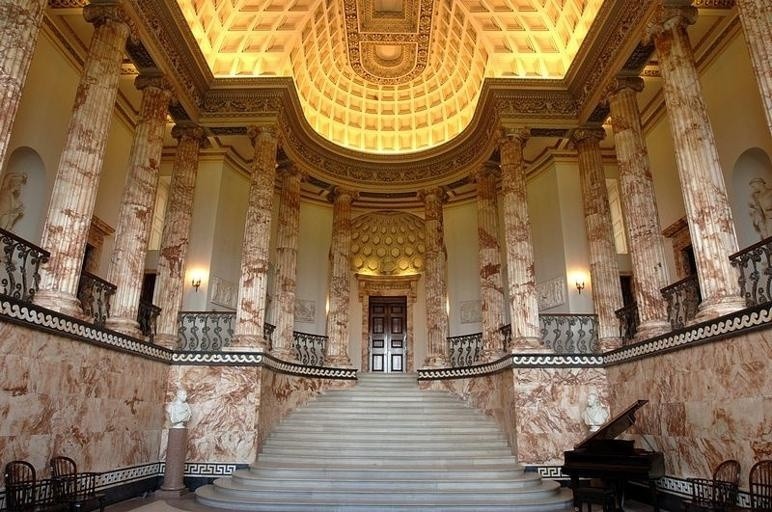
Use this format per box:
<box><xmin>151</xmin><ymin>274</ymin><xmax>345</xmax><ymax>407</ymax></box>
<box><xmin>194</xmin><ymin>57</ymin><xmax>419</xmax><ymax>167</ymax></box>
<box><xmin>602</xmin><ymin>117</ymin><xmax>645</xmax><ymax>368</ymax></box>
<box><xmin>561</xmin><ymin>399</ymin><xmax>665</xmax><ymax>511</ymax></box>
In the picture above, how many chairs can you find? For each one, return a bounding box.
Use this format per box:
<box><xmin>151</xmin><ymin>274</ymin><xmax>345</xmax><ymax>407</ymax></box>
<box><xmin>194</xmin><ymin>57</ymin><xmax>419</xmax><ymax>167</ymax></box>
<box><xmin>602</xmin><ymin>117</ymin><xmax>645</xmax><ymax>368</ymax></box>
<box><xmin>741</xmin><ymin>459</ymin><xmax>772</xmax><ymax>512</ymax></box>
<box><xmin>686</xmin><ymin>460</ymin><xmax>740</xmax><ymax>512</ymax></box>
<box><xmin>5</xmin><ymin>461</ymin><xmax>52</xmax><ymax>512</ymax></box>
<box><xmin>49</xmin><ymin>456</ymin><xmax>106</xmax><ymax>512</ymax></box>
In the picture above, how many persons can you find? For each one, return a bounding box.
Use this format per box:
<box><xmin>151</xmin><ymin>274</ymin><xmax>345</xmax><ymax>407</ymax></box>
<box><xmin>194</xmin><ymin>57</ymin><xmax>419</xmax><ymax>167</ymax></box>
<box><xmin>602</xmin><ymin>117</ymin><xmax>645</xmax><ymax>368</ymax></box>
<box><xmin>749</xmin><ymin>177</ymin><xmax>772</xmax><ymax>237</ymax></box>
<box><xmin>169</xmin><ymin>389</ymin><xmax>192</xmax><ymax>427</ymax></box>
<box><xmin>0</xmin><ymin>173</ymin><xmax>23</xmax><ymax>232</ymax></box>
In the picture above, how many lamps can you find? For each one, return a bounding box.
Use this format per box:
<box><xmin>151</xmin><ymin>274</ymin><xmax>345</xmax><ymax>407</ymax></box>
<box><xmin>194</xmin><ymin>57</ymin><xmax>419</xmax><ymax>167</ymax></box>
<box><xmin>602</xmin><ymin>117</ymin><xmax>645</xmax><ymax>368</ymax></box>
<box><xmin>575</xmin><ymin>281</ymin><xmax>584</xmax><ymax>294</ymax></box>
<box><xmin>192</xmin><ymin>279</ymin><xmax>201</xmax><ymax>292</ymax></box>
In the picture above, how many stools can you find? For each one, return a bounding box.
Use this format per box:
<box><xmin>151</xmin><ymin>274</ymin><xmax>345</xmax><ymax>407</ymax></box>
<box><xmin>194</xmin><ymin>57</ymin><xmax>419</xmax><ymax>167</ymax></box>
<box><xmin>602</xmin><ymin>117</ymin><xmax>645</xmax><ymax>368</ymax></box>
<box><xmin>575</xmin><ymin>488</ymin><xmax>612</xmax><ymax>512</ymax></box>
